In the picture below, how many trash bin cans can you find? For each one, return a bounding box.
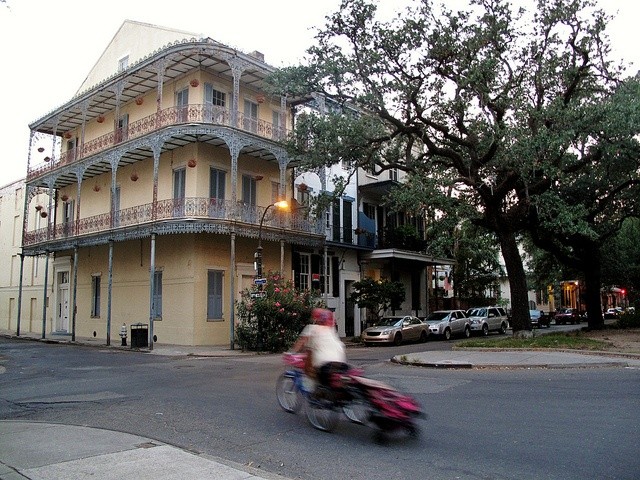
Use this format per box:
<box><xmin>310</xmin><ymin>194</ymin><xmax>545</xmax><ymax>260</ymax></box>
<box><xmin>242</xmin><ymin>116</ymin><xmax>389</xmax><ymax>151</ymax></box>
<box><xmin>131</xmin><ymin>323</ymin><xmax>149</xmax><ymax>349</ymax></box>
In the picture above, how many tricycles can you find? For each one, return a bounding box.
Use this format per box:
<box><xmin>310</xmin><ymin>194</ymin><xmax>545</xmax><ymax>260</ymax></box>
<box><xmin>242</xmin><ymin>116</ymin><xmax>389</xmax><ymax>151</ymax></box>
<box><xmin>275</xmin><ymin>348</ymin><xmax>379</xmax><ymax>432</ymax></box>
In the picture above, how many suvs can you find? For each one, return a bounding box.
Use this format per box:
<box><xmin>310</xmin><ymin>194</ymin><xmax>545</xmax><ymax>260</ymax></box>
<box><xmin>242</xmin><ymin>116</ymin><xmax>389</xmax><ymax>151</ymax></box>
<box><xmin>554</xmin><ymin>307</ymin><xmax>581</xmax><ymax>325</ymax></box>
<box><xmin>423</xmin><ymin>310</ymin><xmax>471</xmax><ymax>340</ymax></box>
<box><xmin>604</xmin><ymin>307</ymin><xmax>621</xmax><ymax>319</ymax></box>
<box><xmin>466</xmin><ymin>305</ymin><xmax>509</xmax><ymax>336</ymax></box>
<box><xmin>360</xmin><ymin>315</ymin><xmax>429</xmax><ymax>345</ymax></box>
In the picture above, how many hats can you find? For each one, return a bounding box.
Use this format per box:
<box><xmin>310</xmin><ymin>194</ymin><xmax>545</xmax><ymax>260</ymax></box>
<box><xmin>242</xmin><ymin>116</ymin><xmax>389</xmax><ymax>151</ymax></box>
<box><xmin>309</xmin><ymin>307</ymin><xmax>334</xmax><ymax>328</ymax></box>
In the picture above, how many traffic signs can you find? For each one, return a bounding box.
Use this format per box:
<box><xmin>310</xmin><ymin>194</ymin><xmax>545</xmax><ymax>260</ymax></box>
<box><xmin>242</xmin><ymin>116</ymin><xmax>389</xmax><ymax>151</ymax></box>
<box><xmin>251</xmin><ymin>290</ymin><xmax>267</xmax><ymax>299</ymax></box>
<box><xmin>254</xmin><ymin>278</ymin><xmax>267</xmax><ymax>284</ymax></box>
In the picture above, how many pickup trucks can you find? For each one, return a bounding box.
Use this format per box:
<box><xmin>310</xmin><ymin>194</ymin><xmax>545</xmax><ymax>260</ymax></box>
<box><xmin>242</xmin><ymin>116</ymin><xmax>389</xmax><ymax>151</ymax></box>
<box><xmin>529</xmin><ymin>310</ymin><xmax>552</xmax><ymax>328</ymax></box>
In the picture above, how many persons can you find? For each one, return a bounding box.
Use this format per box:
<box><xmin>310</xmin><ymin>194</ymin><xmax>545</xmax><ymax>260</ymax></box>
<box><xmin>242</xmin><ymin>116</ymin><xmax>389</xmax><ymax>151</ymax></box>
<box><xmin>293</xmin><ymin>306</ymin><xmax>351</xmax><ymax>405</ymax></box>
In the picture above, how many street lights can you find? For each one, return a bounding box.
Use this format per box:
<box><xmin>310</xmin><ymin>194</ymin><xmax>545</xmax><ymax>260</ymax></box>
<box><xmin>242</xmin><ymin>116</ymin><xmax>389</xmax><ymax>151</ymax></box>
<box><xmin>253</xmin><ymin>201</ymin><xmax>288</xmax><ymax>352</ymax></box>
